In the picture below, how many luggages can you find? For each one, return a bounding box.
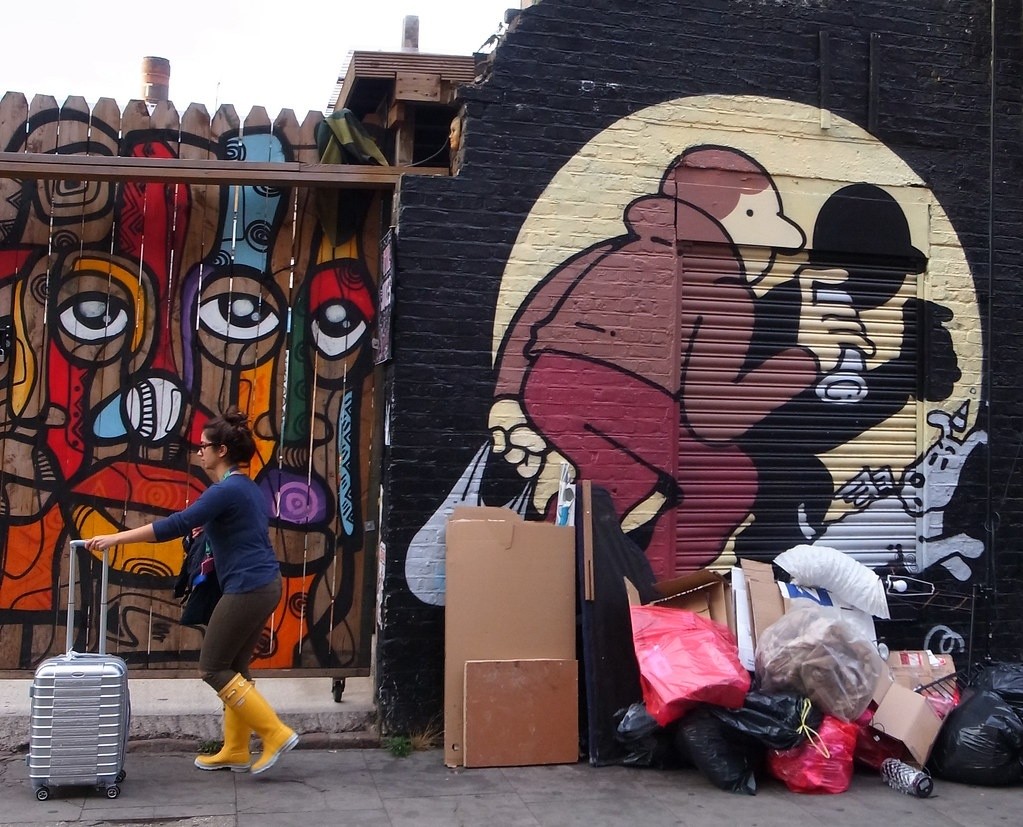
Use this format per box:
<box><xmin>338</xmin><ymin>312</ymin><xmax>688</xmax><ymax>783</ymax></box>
<box><xmin>26</xmin><ymin>541</ymin><xmax>132</xmax><ymax>801</ymax></box>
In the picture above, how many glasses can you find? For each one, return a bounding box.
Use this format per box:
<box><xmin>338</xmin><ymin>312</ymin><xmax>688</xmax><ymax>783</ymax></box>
<box><xmin>198</xmin><ymin>443</ymin><xmax>214</xmax><ymax>451</ymax></box>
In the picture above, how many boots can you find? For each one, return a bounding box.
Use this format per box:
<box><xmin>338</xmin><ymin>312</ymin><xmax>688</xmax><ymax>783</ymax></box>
<box><xmin>194</xmin><ymin>680</ymin><xmax>256</xmax><ymax>773</ymax></box>
<box><xmin>216</xmin><ymin>671</ymin><xmax>300</xmax><ymax>774</ymax></box>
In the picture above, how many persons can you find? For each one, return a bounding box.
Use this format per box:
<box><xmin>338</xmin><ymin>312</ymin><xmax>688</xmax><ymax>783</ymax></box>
<box><xmin>85</xmin><ymin>406</ymin><xmax>300</xmax><ymax>776</ymax></box>
